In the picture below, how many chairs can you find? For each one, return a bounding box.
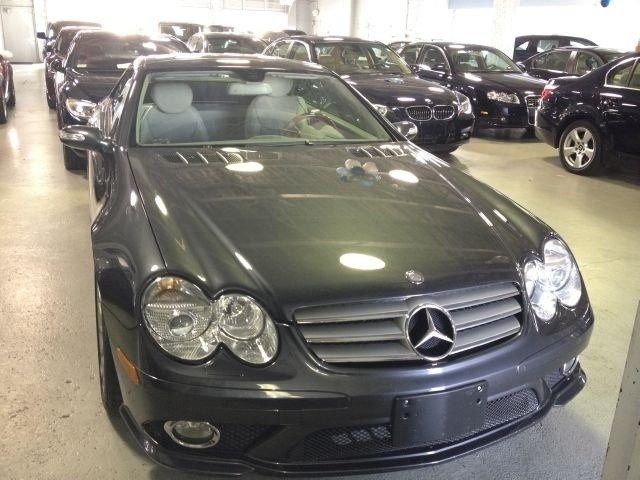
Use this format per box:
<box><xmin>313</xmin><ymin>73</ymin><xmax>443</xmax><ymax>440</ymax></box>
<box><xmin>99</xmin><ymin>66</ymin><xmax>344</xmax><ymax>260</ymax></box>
<box><xmin>139</xmin><ymin>83</ymin><xmax>209</xmax><ymax>143</ymax></box>
<box><xmin>245</xmin><ymin>72</ymin><xmax>309</xmax><ymax>139</ymax></box>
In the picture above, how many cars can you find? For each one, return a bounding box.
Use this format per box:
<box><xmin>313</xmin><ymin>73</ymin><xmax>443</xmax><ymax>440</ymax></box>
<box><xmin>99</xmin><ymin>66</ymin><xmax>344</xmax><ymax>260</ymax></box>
<box><xmin>56</xmin><ymin>51</ymin><xmax>594</xmax><ymax>480</ymax></box>
<box><xmin>0</xmin><ymin>51</ymin><xmax>16</xmax><ymax>124</ymax></box>
